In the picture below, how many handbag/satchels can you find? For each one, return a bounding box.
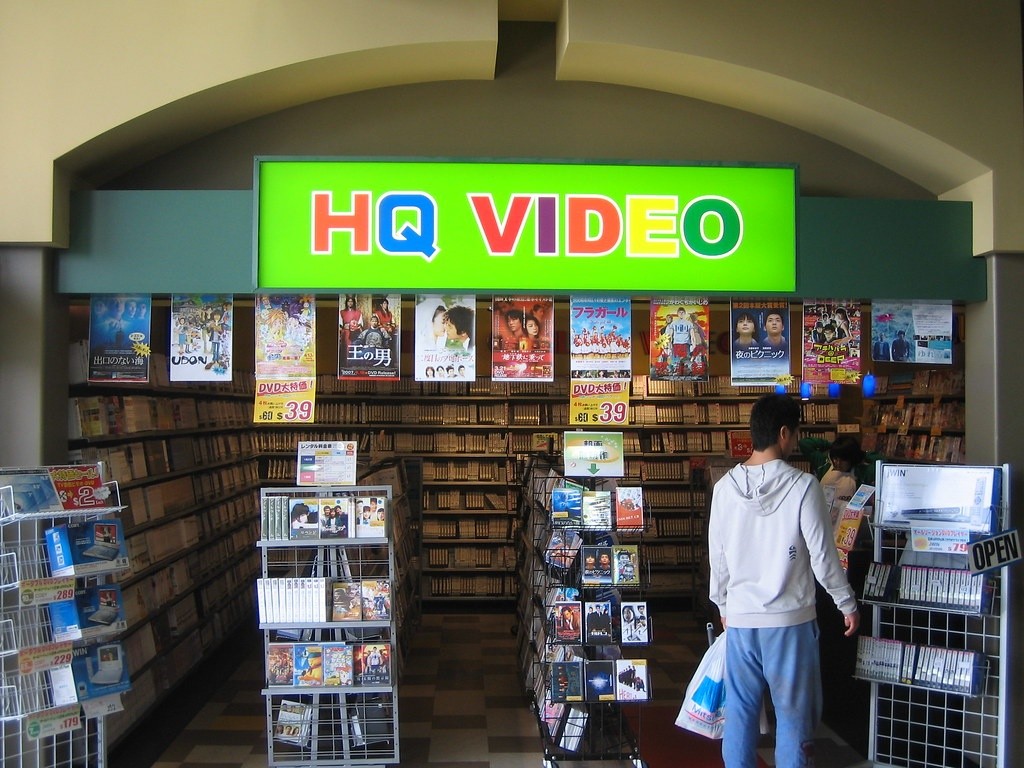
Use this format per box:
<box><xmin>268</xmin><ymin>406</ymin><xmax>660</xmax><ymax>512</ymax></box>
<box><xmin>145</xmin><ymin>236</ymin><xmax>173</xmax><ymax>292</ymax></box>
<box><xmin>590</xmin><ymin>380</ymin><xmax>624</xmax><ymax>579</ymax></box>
<box><xmin>674</xmin><ymin>623</ymin><xmax>769</xmax><ymax>740</ymax></box>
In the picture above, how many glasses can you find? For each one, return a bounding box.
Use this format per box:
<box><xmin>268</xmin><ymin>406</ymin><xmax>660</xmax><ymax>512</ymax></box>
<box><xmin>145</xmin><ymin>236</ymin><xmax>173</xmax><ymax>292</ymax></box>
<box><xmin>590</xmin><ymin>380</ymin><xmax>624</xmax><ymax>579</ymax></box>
<box><xmin>824</xmin><ymin>331</ymin><xmax>832</xmax><ymax>335</ymax></box>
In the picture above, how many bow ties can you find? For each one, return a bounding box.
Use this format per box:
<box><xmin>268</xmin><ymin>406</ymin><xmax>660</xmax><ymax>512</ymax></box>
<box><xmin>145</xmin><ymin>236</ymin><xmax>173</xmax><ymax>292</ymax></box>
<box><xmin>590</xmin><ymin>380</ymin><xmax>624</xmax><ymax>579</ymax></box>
<box><xmin>307</xmin><ymin>664</ymin><xmax>321</xmax><ymax>675</ymax></box>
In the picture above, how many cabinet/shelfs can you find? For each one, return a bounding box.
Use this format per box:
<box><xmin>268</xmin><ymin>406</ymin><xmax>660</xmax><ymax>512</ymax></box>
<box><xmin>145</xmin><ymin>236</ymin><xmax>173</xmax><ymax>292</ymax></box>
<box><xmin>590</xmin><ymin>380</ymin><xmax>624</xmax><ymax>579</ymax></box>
<box><xmin>0</xmin><ymin>390</ymin><xmax>1019</xmax><ymax>768</ymax></box>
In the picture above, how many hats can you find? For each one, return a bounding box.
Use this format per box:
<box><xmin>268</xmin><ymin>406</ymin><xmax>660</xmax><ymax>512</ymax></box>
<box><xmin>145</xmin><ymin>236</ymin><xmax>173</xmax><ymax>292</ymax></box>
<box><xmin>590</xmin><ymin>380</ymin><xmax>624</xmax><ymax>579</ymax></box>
<box><xmin>305</xmin><ymin>652</ymin><xmax>321</xmax><ymax>658</ymax></box>
<box><xmin>815</xmin><ymin>322</ymin><xmax>825</xmax><ymax>329</ymax></box>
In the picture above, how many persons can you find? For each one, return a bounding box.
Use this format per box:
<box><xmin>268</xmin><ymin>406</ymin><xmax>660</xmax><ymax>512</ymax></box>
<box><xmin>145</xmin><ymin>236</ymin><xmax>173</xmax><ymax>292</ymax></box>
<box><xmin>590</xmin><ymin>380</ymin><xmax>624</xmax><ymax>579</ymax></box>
<box><xmin>812</xmin><ymin>305</ymin><xmax>853</xmax><ymax>357</ymax></box>
<box><xmin>618</xmin><ymin>664</ymin><xmax>646</xmax><ymax>693</ymax></box>
<box><xmin>733</xmin><ymin>309</ymin><xmax>787</xmax><ymax>348</ymax></box>
<box><xmin>320</xmin><ymin>504</ymin><xmax>349</xmax><ymax>536</ymax></box>
<box><xmin>363</xmin><ymin>646</ymin><xmax>389</xmax><ymax>673</ymax></box>
<box><xmin>873</xmin><ymin>328</ymin><xmax>910</xmax><ymax>361</ymax></box>
<box><xmin>95</xmin><ymin>297</ymin><xmax>147</xmax><ymax>356</ymax></box>
<box><xmin>587</xmin><ymin>605</ymin><xmax>611</xmax><ymax>635</ymax></box>
<box><xmin>357</xmin><ymin>498</ymin><xmax>385</xmax><ymax>528</ymax></box>
<box><xmin>340</xmin><ymin>296</ymin><xmax>397</xmax><ymax>368</ymax></box>
<box><xmin>584</xmin><ymin>552</ymin><xmax>610</xmax><ymax>576</ymax></box>
<box><xmin>708</xmin><ymin>393</ymin><xmax>862</xmax><ymax>768</ymax></box>
<box><xmin>798</xmin><ymin>430</ymin><xmax>886</xmax><ymax>683</ymax></box>
<box><xmin>504</xmin><ymin>304</ymin><xmax>546</xmax><ymax>339</ymax></box>
<box><xmin>623</xmin><ymin>603</ymin><xmax>648</xmax><ymax>636</ymax></box>
<box><xmin>416</xmin><ymin>298</ymin><xmax>473</xmax><ymax>380</ymax></box>
<box><xmin>561</xmin><ymin>607</ymin><xmax>580</xmax><ymax>632</ymax></box>
